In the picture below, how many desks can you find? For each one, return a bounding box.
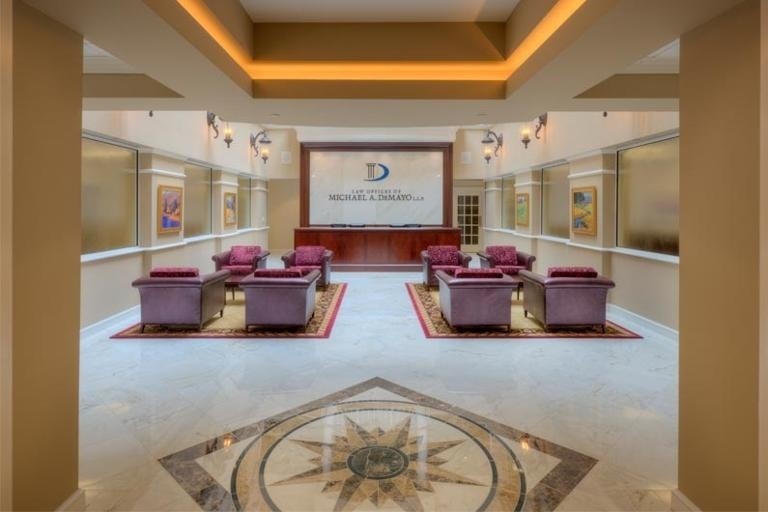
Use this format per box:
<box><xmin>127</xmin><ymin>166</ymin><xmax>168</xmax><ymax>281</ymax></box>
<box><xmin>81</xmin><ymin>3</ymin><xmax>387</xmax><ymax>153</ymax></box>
<box><xmin>510</xmin><ymin>275</ymin><xmax>523</xmax><ymax>300</ymax></box>
<box><xmin>224</xmin><ymin>276</ymin><xmax>242</xmax><ymax>305</ymax></box>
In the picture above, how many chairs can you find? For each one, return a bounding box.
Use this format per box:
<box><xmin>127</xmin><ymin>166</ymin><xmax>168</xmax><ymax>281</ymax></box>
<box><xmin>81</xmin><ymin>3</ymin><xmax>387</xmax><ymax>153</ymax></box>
<box><xmin>279</xmin><ymin>244</ymin><xmax>335</xmax><ymax>292</ymax></box>
<box><xmin>131</xmin><ymin>264</ymin><xmax>233</xmax><ymax>336</ymax></box>
<box><xmin>475</xmin><ymin>242</ymin><xmax>535</xmax><ymax>292</ymax></box>
<box><xmin>212</xmin><ymin>244</ymin><xmax>271</xmax><ymax>291</ymax></box>
<box><xmin>431</xmin><ymin>263</ymin><xmax>522</xmax><ymax>332</ymax></box>
<box><xmin>515</xmin><ymin>263</ymin><xmax>617</xmax><ymax>334</ymax></box>
<box><xmin>419</xmin><ymin>244</ymin><xmax>471</xmax><ymax>294</ymax></box>
<box><xmin>234</xmin><ymin>263</ymin><xmax>324</xmax><ymax>335</ymax></box>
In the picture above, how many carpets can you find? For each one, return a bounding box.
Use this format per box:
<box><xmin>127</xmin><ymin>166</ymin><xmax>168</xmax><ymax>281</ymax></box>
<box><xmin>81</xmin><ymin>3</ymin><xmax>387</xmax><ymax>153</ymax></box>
<box><xmin>107</xmin><ymin>279</ymin><xmax>349</xmax><ymax>338</ymax></box>
<box><xmin>400</xmin><ymin>278</ymin><xmax>646</xmax><ymax>340</ymax></box>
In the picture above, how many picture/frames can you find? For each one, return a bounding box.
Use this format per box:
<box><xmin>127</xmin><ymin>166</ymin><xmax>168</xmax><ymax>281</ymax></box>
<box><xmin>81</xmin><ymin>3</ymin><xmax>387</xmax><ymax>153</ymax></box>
<box><xmin>156</xmin><ymin>182</ymin><xmax>185</xmax><ymax>236</ymax></box>
<box><xmin>220</xmin><ymin>190</ymin><xmax>240</xmax><ymax>228</ymax></box>
<box><xmin>513</xmin><ymin>191</ymin><xmax>531</xmax><ymax>229</ymax></box>
<box><xmin>569</xmin><ymin>183</ymin><xmax>600</xmax><ymax>239</ymax></box>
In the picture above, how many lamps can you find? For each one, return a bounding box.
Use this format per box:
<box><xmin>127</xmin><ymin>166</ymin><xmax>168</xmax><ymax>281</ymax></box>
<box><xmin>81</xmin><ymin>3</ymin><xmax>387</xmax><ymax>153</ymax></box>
<box><xmin>518</xmin><ymin>110</ymin><xmax>550</xmax><ymax>150</ymax></box>
<box><xmin>479</xmin><ymin>125</ymin><xmax>507</xmax><ymax>166</ymax></box>
<box><xmin>204</xmin><ymin>108</ymin><xmax>239</xmax><ymax>149</ymax></box>
<box><xmin>245</xmin><ymin>127</ymin><xmax>273</xmax><ymax>166</ymax></box>
